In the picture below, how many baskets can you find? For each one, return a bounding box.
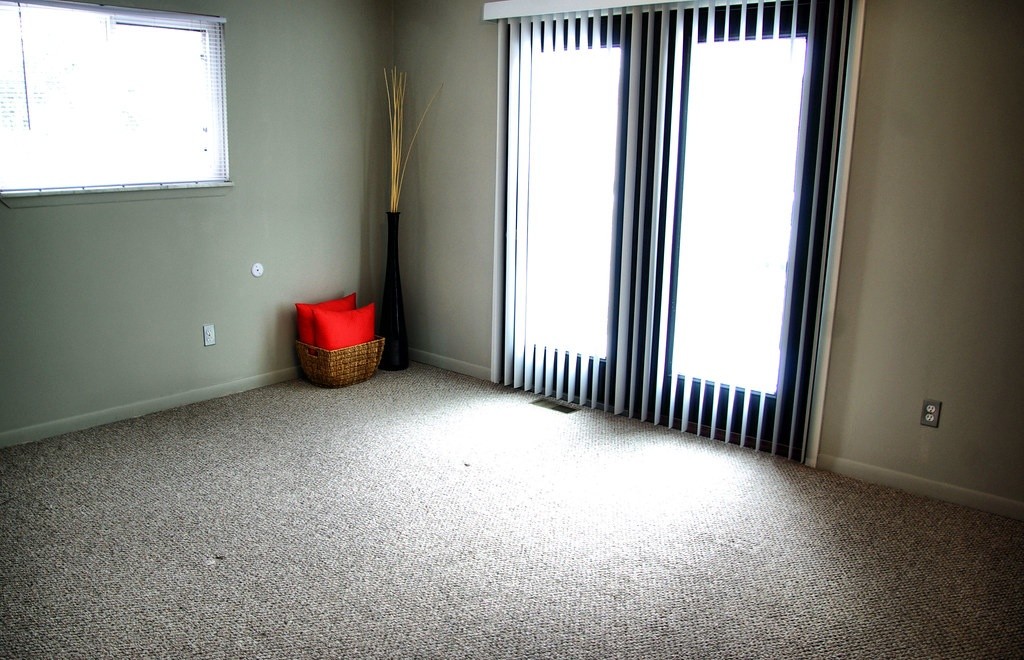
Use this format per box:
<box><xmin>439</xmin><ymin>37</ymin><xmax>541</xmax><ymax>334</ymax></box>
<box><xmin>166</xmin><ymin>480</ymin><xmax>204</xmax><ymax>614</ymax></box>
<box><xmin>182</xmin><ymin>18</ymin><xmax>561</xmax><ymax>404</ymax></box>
<box><xmin>294</xmin><ymin>334</ymin><xmax>387</xmax><ymax>388</ymax></box>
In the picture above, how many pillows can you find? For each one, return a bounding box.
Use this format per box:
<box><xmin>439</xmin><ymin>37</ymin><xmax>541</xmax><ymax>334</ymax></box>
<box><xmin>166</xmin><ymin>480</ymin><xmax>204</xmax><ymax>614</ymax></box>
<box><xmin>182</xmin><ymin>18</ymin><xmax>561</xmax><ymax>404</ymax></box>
<box><xmin>311</xmin><ymin>303</ymin><xmax>374</xmax><ymax>350</ymax></box>
<box><xmin>295</xmin><ymin>293</ymin><xmax>356</xmax><ymax>347</ymax></box>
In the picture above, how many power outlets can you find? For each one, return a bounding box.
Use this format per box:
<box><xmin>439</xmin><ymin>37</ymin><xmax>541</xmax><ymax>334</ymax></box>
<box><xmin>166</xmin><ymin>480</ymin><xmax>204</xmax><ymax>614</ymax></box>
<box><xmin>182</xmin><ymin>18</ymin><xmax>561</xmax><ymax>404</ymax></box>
<box><xmin>252</xmin><ymin>263</ymin><xmax>263</xmax><ymax>276</ymax></box>
<box><xmin>203</xmin><ymin>324</ymin><xmax>215</xmax><ymax>345</ymax></box>
<box><xmin>919</xmin><ymin>399</ymin><xmax>942</xmax><ymax>428</ymax></box>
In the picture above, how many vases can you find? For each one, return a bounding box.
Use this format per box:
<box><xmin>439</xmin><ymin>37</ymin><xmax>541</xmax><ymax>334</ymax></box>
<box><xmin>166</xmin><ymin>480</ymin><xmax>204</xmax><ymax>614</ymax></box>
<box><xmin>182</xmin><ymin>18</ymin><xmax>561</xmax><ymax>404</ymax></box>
<box><xmin>379</xmin><ymin>212</ymin><xmax>410</xmax><ymax>372</ymax></box>
